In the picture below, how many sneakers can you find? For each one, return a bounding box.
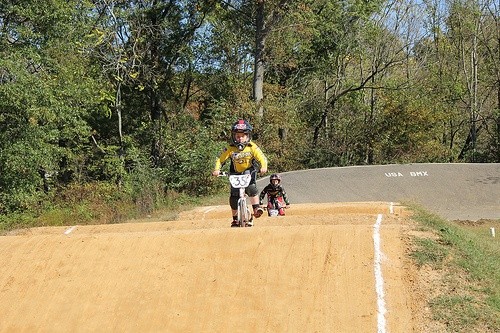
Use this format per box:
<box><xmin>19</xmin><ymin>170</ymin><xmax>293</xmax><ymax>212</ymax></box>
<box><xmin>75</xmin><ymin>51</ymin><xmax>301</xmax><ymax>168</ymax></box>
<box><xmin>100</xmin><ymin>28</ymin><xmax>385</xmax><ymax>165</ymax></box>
<box><xmin>232</xmin><ymin>220</ymin><xmax>239</xmax><ymax>226</ymax></box>
<box><xmin>254</xmin><ymin>207</ymin><xmax>263</xmax><ymax>218</ymax></box>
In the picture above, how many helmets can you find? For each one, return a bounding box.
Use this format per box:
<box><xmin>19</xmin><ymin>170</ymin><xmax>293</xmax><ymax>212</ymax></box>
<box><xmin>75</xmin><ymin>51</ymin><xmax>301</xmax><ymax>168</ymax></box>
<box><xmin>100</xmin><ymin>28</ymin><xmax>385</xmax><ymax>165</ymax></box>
<box><xmin>270</xmin><ymin>173</ymin><xmax>281</xmax><ymax>188</ymax></box>
<box><xmin>231</xmin><ymin>120</ymin><xmax>253</xmax><ymax>150</ymax></box>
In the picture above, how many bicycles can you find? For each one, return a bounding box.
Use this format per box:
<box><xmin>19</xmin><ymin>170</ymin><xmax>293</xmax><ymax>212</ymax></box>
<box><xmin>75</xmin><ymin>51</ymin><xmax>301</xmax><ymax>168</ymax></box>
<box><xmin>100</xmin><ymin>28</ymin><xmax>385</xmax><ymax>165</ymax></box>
<box><xmin>259</xmin><ymin>205</ymin><xmax>291</xmax><ymax>217</ymax></box>
<box><xmin>211</xmin><ymin>169</ymin><xmax>263</xmax><ymax>227</ymax></box>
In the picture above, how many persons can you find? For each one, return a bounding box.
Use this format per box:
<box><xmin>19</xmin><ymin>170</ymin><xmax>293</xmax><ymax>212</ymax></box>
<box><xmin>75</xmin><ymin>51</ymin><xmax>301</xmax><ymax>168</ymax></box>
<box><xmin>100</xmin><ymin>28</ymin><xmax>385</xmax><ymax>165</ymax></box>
<box><xmin>212</xmin><ymin>120</ymin><xmax>268</xmax><ymax>227</ymax></box>
<box><xmin>259</xmin><ymin>173</ymin><xmax>290</xmax><ymax>216</ymax></box>
